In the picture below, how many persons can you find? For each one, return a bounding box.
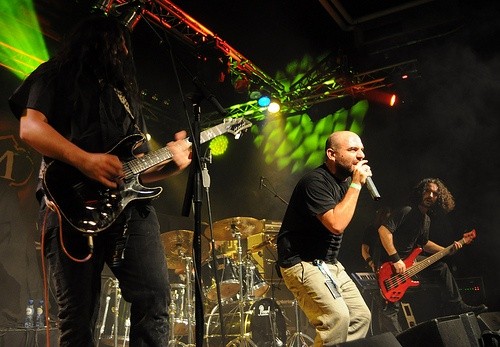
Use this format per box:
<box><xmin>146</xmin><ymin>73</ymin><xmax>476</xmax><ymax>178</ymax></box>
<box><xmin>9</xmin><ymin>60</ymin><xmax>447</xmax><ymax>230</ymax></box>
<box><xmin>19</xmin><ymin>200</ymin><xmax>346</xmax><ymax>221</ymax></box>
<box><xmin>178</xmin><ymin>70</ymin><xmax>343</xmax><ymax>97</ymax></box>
<box><xmin>278</xmin><ymin>131</ymin><xmax>372</xmax><ymax>347</ymax></box>
<box><xmin>19</xmin><ymin>12</ymin><xmax>193</xmax><ymax>347</ymax></box>
<box><xmin>362</xmin><ymin>177</ymin><xmax>487</xmax><ymax>334</ymax></box>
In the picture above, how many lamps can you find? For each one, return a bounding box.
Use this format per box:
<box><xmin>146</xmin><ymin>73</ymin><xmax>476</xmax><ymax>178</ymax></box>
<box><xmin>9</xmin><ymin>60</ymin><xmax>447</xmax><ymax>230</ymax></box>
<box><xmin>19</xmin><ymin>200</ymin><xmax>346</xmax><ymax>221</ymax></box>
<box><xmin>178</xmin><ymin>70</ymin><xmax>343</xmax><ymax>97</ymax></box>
<box><xmin>92</xmin><ymin>0</ymin><xmax>146</xmax><ymax>31</ymax></box>
<box><xmin>247</xmin><ymin>83</ymin><xmax>281</xmax><ymax>113</ymax></box>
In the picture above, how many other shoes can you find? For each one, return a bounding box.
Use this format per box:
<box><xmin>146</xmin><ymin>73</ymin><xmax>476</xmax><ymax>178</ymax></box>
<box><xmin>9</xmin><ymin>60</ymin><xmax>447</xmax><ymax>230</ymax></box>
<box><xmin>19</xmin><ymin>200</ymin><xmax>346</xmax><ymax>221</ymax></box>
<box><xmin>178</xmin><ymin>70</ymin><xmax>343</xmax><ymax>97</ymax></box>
<box><xmin>451</xmin><ymin>301</ymin><xmax>488</xmax><ymax>315</ymax></box>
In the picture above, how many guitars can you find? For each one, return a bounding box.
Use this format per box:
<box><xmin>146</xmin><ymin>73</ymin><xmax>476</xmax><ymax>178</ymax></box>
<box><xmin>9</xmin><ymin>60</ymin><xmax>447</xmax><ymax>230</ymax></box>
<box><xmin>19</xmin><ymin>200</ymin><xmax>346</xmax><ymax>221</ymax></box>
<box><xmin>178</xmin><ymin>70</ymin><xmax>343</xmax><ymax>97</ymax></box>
<box><xmin>38</xmin><ymin>114</ymin><xmax>254</xmax><ymax>242</ymax></box>
<box><xmin>375</xmin><ymin>228</ymin><xmax>476</xmax><ymax>303</ymax></box>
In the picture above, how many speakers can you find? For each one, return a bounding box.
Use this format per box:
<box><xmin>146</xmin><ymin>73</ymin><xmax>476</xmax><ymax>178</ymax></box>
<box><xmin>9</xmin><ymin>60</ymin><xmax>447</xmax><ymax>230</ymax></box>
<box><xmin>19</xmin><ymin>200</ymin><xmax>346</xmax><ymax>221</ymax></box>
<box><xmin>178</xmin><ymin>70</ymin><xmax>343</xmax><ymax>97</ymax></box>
<box><xmin>325</xmin><ymin>311</ymin><xmax>500</xmax><ymax>347</ymax></box>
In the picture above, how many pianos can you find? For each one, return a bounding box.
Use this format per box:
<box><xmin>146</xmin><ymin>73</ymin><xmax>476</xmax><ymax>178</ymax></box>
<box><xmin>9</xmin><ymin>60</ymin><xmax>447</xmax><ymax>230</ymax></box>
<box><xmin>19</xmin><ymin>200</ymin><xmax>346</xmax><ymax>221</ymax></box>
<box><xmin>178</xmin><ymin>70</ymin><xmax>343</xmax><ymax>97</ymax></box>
<box><xmin>351</xmin><ymin>269</ymin><xmax>421</xmax><ymax>292</ymax></box>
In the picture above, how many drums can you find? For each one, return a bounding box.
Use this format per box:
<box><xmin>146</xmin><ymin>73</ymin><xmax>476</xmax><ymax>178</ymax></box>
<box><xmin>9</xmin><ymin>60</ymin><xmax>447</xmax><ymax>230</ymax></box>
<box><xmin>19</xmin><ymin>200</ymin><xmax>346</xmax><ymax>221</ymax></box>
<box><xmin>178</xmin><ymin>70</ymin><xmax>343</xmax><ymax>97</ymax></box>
<box><xmin>98</xmin><ymin>279</ymin><xmax>132</xmax><ymax>346</ymax></box>
<box><xmin>168</xmin><ymin>283</ymin><xmax>195</xmax><ymax>336</ymax></box>
<box><xmin>236</xmin><ymin>261</ymin><xmax>270</xmax><ymax>298</ymax></box>
<box><xmin>206</xmin><ymin>296</ymin><xmax>287</xmax><ymax>347</ymax></box>
<box><xmin>199</xmin><ymin>254</ymin><xmax>242</xmax><ymax>302</ymax></box>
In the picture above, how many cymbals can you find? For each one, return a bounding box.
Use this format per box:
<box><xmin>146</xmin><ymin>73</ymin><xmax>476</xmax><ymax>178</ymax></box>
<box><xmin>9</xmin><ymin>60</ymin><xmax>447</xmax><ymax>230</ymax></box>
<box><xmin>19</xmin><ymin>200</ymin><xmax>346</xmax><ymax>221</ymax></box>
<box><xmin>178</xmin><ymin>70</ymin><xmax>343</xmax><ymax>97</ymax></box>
<box><xmin>203</xmin><ymin>217</ymin><xmax>263</xmax><ymax>242</ymax></box>
<box><xmin>160</xmin><ymin>230</ymin><xmax>211</xmax><ymax>270</ymax></box>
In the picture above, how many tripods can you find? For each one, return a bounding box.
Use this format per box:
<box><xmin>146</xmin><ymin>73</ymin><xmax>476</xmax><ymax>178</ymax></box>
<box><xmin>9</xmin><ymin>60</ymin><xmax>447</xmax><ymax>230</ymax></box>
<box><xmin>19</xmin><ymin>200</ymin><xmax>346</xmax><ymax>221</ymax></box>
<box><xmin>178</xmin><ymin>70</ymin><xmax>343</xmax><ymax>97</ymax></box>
<box><xmin>162</xmin><ymin>226</ymin><xmax>313</xmax><ymax>347</ymax></box>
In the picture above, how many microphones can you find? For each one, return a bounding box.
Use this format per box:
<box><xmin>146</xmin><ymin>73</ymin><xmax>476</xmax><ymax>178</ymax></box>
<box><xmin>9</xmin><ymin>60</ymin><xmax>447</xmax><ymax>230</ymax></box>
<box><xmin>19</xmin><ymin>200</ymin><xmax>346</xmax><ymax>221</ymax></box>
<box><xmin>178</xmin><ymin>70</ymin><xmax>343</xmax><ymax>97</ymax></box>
<box><xmin>275</xmin><ymin>337</ymin><xmax>284</xmax><ymax>347</ymax></box>
<box><xmin>365</xmin><ymin>176</ymin><xmax>381</xmax><ymax>200</ymax></box>
<box><xmin>260</xmin><ymin>176</ymin><xmax>264</xmax><ymax>189</ymax></box>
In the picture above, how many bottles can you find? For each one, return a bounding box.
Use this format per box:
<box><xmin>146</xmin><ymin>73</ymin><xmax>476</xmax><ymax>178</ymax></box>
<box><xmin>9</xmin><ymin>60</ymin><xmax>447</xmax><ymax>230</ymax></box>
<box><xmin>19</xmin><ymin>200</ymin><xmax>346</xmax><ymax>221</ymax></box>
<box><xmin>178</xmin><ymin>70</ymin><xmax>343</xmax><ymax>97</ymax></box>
<box><xmin>36</xmin><ymin>300</ymin><xmax>44</xmax><ymax>329</ymax></box>
<box><xmin>25</xmin><ymin>299</ymin><xmax>34</xmax><ymax>328</ymax></box>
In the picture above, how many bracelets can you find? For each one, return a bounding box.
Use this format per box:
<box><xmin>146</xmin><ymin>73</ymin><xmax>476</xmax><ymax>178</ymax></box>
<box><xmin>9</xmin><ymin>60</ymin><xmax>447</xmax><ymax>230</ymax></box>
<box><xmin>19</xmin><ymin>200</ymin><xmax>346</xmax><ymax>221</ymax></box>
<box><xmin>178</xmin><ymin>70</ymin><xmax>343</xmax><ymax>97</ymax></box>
<box><xmin>365</xmin><ymin>256</ymin><xmax>373</xmax><ymax>262</ymax></box>
<box><xmin>390</xmin><ymin>253</ymin><xmax>401</xmax><ymax>263</ymax></box>
<box><xmin>349</xmin><ymin>183</ymin><xmax>362</xmax><ymax>191</ymax></box>
